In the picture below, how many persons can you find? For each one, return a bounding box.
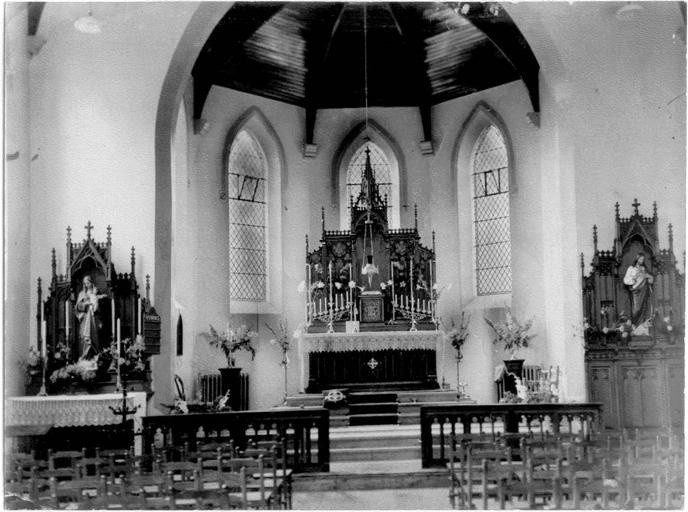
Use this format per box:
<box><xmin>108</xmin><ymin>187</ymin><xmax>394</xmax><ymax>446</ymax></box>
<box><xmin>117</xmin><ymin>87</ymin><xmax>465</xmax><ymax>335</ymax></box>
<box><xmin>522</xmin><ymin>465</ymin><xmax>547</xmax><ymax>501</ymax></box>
<box><xmin>623</xmin><ymin>252</ymin><xmax>654</xmax><ymax>323</ymax></box>
<box><xmin>74</xmin><ymin>275</ymin><xmax>102</xmax><ymax>360</ymax></box>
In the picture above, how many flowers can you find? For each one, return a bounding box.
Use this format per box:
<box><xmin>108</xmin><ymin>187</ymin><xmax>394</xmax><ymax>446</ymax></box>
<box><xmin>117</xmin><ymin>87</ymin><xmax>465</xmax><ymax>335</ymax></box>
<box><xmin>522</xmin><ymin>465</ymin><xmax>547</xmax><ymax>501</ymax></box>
<box><xmin>433</xmin><ymin>310</ymin><xmax>474</xmax><ymax>350</ymax></box>
<box><xmin>265</xmin><ymin>318</ymin><xmax>291</xmax><ymax>351</ymax></box>
<box><xmin>483</xmin><ymin>301</ymin><xmax>537</xmax><ymax>350</ymax></box>
<box><xmin>198</xmin><ymin>320</ymin><xmax>257</xmax><ymax>361</ymax></box>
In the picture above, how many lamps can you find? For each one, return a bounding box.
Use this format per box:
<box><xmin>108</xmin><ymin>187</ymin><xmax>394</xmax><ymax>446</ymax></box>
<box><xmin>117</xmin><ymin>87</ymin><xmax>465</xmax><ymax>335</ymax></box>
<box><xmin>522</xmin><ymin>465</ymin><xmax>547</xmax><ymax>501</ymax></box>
<box><xmin>360</xmin><ymin>2</ymin><xmax>380</xmax><ymax>287</ymax></box>
<box><xmin>615</xmin><ymin>1</ymin><xmax>646</xmax><ymax>22</ymax></box>
<box><xmin>74</xmin><ymin>2</ymin><xmax>102</xmax><ymax>35</ymax></box>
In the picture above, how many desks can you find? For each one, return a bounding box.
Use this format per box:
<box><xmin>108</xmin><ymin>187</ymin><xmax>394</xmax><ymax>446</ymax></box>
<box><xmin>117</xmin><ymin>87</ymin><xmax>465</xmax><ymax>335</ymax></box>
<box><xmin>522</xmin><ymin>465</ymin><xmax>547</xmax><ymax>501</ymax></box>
<box><xmin>4</xmin><ymin>391</ymin><xmax>148</xmax><ymax>475</ymax></box>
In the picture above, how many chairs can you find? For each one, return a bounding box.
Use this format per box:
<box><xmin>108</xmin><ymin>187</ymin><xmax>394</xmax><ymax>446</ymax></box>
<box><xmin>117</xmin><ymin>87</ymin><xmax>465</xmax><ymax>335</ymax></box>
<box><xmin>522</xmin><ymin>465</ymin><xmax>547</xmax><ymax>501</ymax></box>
<box><xmin>444</xmin><ymin>421</ymin><xmax>684</xmax><ymax>509</ymax></box>
<box><xmin>4</xmin><ymin>433</ymin><xmax>295</xmax><ymax>510</ymax></box>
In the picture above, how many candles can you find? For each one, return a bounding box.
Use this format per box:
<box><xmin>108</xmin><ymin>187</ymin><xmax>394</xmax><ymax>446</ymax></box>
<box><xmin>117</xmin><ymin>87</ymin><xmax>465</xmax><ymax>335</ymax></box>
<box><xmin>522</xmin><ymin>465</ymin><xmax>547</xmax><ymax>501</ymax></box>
<box><xmin>137</xmin><ymin>297</ymin><xmax>142</xmax><ymax>334</ymax></box>
<box><xmin>111</xmin><ymin>298</ymin><xmax>115</xmax><ymax>338</ymax></box>
<box><xmin>40</xmin><ymin>300</ymin><xmax>45</xmax><ymax>341</ymax></box>
<box><xmin>309</xmin><ymin>290</ymin><xmax>350</xmax><ymax>316</ymax></box>
<box><xmin>117</xmin><ymin>316</ymin><xmax>122</xmax><ymax>355</ymax></box>
<box><xmin>393</xmin><ymin>294</ymin><xmax>433</xmax><ymax>316</ymax></box>
<box><xmin>41</xmin><ymin>319</ymin><xmax>47</xmax><ymax>360</ymax></box>
<box><xmin>65</xmin><ymin>300</ymin><xmax>70</xmax><ymax>340</ymax></box>
